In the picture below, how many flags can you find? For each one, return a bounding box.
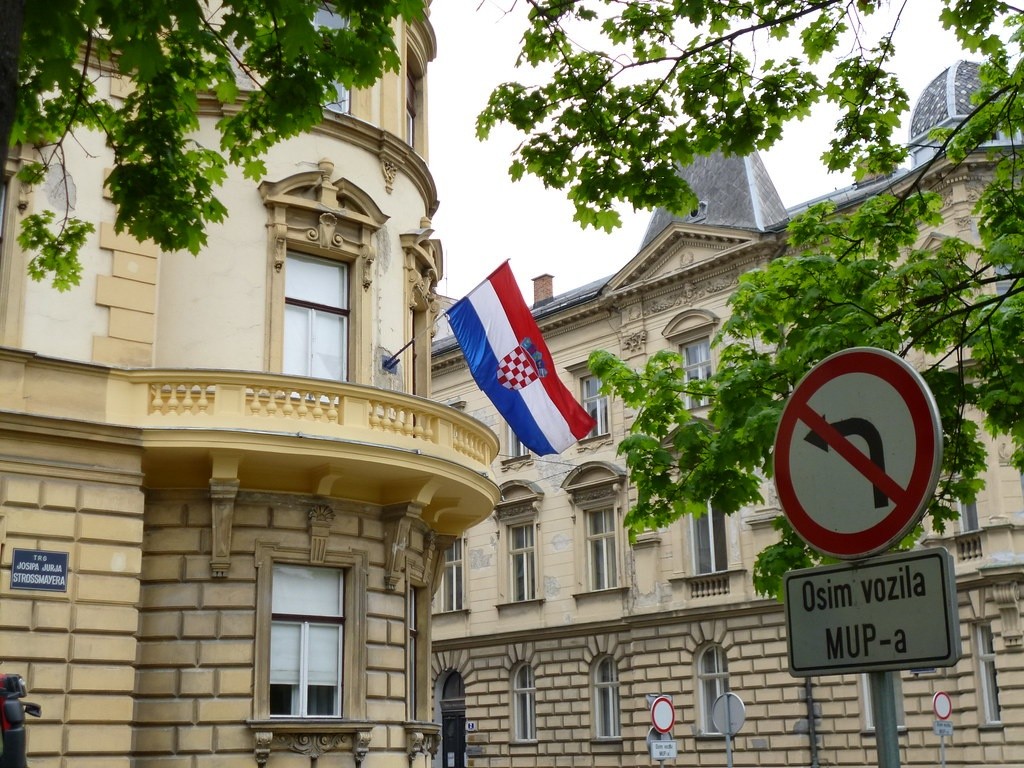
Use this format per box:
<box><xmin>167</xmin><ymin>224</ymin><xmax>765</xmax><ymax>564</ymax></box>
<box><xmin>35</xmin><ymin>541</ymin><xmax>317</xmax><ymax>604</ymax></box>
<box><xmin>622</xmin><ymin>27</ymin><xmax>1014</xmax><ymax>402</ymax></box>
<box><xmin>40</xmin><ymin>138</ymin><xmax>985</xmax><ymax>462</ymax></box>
<box><xmin>444</xmin><ymin>260</ymin><xmax>598</xmax><ymax>457</ymax></box>
